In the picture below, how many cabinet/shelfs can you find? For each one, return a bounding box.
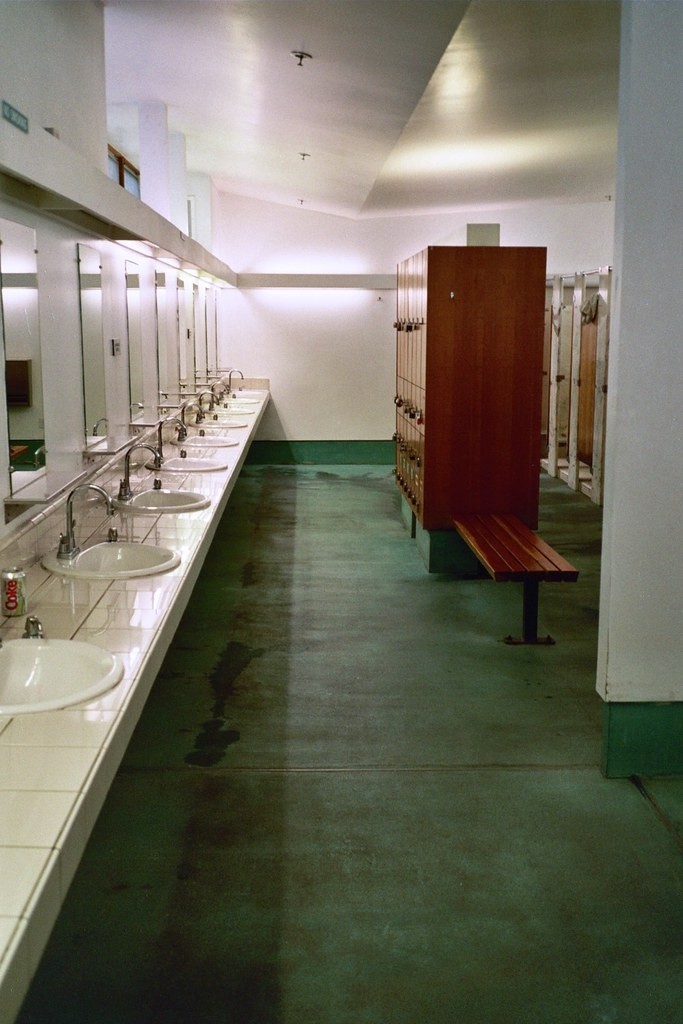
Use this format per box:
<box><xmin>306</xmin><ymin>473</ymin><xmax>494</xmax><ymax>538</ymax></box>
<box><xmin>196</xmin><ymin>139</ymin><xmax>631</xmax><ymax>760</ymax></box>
<box><xmin>393</xmin><ymin>246</ymin><xmax>548</xmax><ymax>573</ymax></box>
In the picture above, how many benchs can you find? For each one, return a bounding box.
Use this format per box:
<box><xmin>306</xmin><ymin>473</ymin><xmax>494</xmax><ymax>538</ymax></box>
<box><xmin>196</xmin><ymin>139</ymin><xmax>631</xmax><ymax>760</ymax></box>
<box><xmin>449</xmin><ymin>512</ymin><xmax>580</xmax><ymax>645</ymax></box>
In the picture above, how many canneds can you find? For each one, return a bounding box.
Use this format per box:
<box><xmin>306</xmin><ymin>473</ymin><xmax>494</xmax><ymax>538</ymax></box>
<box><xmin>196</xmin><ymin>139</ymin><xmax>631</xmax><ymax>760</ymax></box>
<box><xmin>0</xmin><ymin>567</ymin><xmax>27</xmax><ymax>616</ymax></box>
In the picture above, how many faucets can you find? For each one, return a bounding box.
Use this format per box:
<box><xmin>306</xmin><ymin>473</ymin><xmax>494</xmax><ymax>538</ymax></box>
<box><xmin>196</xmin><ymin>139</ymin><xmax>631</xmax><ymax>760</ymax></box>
<box><xmin>32</xmin><ymin>445</ymin><xmax>45</xmax><ymax>471</ymax></box>
<box><xmin>153</xmin><ymin>417</ymin><xmax>186</xmax><ymax>464</ymax></box>
<box><xmin>174</xmin><ymin>403</ymin><xmax>203</xmax><ymax>442</ymax></box>
<box><xmin>225</xmin><ymin>369</ymin><xmax>243</xmax><ymax>395</ymax></box>
<box><xmin>208</xmin><ymin>381</ymin><xmax>230</xmax><ymax>411</ymax></box>
<box><xmin>60</xmin><ymin>577</ymin><xmax>114</xmax><ymax>637</ymax></box>
<box><xmin>130</xmin><ymin>402</ymin><xmax>143</xmax><ymax>407</ymax></box>
<box><xmin>117</xmin><ymin>443</ymin><xmax>160</xmax><ymax>501</ymax></box>
<box><xmin>55</xmin><ymin>483</ymin><xmax>114</xmax><ymax>561</ymax></box>
<box><xmin>91</xmin><ymin>417</ymin><xmax>109</xmax><ymax>436</ymax></box>
<box><xmin>194</xmin><ymin>391</ymin><xmax>219</xmax><ymax>425</ymax></box>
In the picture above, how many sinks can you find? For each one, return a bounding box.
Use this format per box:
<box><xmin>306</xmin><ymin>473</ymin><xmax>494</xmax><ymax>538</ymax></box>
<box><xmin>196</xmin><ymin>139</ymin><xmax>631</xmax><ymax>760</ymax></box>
<box><xmin>40</xmin><ymin>541</ymin><xmax>181</xmax><ymax>579</ymax></box>
<box><xmin>0</xmin><ymin>639</ymin><xmax>124</xmax><ymax>715</ymax></box>
<box><xmin>200</xmin><ymin>408</ymin><xmax>255</xmax><ymax>415</ymax></box>
<box><xmin>144</xmin><ymin>458</ymin><xmax>228</xmax><ymax>472</ymax></box>
<box><xmin>188</xmin><ymin>420</ymin><xmax>248</xmax><ymax>428</ymax></box>
<box><xmin>224</xmin><ymin>390</ymin><xmax>265</xmax><ymax>396</ymax></box>
<box><xmin>214</xmin><ymin>398</ymin><xmax>260</xmax><ymax>404</ymax></box>
<box><xmin>111</xmin><ymin>489</ymin><xmax>211</xmax><ymax>512</ymax></box>
<box><xmin>169</xmin><ymin>436</ymin><xmax>239</xmax><ymax>447</ymax></box>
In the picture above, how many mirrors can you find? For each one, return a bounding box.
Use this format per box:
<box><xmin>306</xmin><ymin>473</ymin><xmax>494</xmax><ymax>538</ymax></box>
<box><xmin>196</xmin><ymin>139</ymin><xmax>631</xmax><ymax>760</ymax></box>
<box><xmin>77</xmin><ymin>243</ymin><xmax>109</xmax><ymax>450</ymax></box>
<box><xmin>0</xmin><ymin>216</ymin><xmax>49</xmax><ymax>495</ymax></box>
<box><xmin>177</xmin><ymin>278</ymin><xmax>220</xmax><ymax>380</ymax></box>
<box><xmin>155</xmin><ymin>268</ymin><xmax>169</xmax><ymax>405</ymax></box>
<box><xmin>125</xmin><ymin>260</ymin><xmax>145</xmax><ymax>423</ymax></box>
